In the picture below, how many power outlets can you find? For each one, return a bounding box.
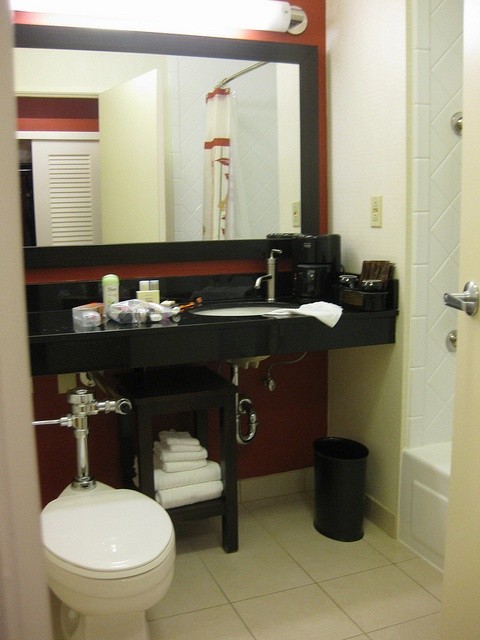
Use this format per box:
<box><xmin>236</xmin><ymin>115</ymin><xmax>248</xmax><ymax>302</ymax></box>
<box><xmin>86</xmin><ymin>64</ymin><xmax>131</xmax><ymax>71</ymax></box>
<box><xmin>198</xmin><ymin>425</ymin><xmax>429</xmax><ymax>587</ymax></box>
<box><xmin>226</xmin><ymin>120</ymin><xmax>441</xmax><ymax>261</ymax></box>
<box><xmin>370</xmin><ymin>195</ymin><xmax>382</xmax><ymax>227</ymax></box>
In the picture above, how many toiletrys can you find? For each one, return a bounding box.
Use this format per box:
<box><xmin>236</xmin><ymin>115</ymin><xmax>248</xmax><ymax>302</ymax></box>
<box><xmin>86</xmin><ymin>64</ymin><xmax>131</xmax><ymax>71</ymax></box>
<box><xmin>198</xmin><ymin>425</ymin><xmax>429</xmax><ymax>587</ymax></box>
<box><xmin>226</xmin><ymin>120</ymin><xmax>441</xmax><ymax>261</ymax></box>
<box><xmin>101</xmin><ymin>274</ymin><xmax>120</xmax><ymax>304</ymax></box>
<box><xmin>138</xmin><ymin>279</ymin><xmax>158</xmax><ymax>290</ymax></box>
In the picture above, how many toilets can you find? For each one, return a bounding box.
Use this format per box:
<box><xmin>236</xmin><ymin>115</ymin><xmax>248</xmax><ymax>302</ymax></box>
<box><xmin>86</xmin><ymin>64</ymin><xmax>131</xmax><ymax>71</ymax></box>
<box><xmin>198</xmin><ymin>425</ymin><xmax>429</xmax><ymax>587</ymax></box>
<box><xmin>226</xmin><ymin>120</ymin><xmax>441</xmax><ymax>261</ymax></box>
<box><xmin>41</xmin><ymin>482</ymin><xmax>177</xmax><ymax>635</ymax></box>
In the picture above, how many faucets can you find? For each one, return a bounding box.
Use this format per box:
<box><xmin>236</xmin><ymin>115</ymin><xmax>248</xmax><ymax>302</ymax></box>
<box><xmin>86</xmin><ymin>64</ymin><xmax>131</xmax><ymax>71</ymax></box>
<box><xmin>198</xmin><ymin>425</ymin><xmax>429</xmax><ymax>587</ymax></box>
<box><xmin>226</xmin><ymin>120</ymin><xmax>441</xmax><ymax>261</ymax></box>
<box><xmin>254</xmin><ymin>249</ymin><xmax>283</xmax><ymax>303</ymax></box>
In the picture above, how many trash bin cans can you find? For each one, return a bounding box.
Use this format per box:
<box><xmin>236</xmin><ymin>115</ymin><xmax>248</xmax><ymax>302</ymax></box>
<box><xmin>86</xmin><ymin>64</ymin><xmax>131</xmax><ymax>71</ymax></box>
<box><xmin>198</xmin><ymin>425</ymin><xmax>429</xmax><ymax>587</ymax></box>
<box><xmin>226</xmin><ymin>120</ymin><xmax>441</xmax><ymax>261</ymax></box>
<box><xmin>312</xmin><ymin>437</ymin><xmax>369</xmax><ymax>542</ymax></box>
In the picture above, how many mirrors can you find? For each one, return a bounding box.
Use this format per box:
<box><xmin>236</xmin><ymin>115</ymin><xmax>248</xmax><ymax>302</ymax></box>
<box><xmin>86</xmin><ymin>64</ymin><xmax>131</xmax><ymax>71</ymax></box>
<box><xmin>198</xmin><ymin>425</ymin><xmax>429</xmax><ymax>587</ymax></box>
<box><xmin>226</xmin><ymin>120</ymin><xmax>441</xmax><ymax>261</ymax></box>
<box><xmin>14</xmin><ymin>23</ymin><xmax>319</xmax><ymax>271</ymax></box>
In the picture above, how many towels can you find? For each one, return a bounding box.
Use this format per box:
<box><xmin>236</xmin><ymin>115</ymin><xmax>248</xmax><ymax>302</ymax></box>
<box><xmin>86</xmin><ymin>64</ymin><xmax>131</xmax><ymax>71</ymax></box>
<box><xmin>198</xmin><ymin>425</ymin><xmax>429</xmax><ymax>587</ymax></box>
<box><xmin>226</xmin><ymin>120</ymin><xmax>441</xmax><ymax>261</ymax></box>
<box><xmin>134</xmin><ymin>426</ymin><xmax>226</xmax><ymax>510</ymax></box>
<box><xmin>268</xmin><ymin>301</ymin><xmax>344</xmax><ymax>328</ymax></box>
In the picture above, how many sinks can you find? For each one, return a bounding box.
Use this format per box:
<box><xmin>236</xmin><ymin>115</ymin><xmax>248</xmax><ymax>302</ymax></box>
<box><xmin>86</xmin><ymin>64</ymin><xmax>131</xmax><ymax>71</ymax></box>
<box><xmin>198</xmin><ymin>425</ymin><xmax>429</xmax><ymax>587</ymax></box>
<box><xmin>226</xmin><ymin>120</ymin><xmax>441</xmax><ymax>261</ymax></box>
<box><xmin>188</xmin><ymin>302</ymin><xmax>295</xmax><ymax>317</ymax></box>
